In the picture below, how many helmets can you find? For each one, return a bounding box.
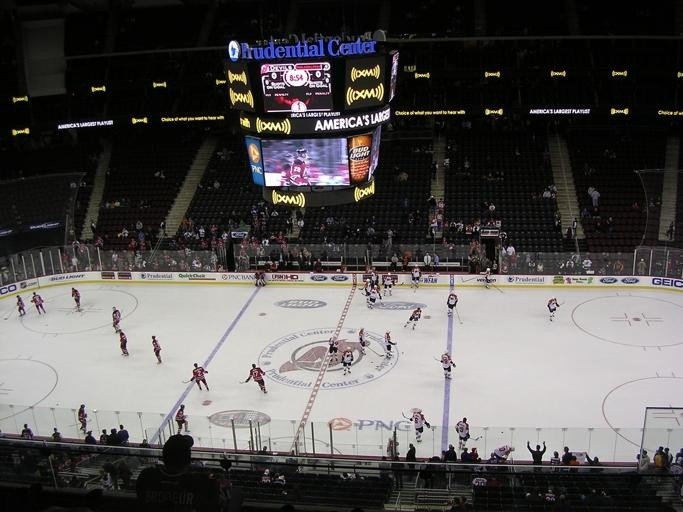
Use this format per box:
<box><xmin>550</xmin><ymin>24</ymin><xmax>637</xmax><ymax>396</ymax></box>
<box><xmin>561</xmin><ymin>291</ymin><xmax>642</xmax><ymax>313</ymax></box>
<box><xmin>295</xmin><ymin>147</ymin><xmax>307</xmax><ymax>153</ymax></box>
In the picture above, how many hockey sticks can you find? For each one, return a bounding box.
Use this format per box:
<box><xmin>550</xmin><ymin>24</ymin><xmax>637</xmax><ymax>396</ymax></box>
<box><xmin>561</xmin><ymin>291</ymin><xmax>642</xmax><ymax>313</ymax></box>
<box><xmin>402</xmin><ymin>411</ymin><xmax>411</xmax><ymax>419</ymax></box>
<box><xmin>455</xmin><ymin>305</ymin><xmax>464</xmax><ymax>325</ymax></box>
<box><xmin>366</xmin><ymin>345</ymin><xmax>384</xmax><ymax>357</ymax></box>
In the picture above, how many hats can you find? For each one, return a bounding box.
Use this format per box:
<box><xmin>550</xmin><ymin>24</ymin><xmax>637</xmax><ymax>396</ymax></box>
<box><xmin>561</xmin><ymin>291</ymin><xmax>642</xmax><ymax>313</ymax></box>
<box><xmin>162</xmin><ymin>434</ymin><xmax>194</xmax><ymax>462</ymax></box>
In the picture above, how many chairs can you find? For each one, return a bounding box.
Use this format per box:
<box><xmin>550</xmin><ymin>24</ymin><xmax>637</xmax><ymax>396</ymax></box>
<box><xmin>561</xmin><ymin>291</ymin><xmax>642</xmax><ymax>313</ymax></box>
<box><xmin>0</xmin><ymin>433</ymin><xmax>683</xmax><ymax>512</ymax></box>
<box><xmin>0</xmin><ymin>129</ymin><xmax>683</xmax><ymax>287</ymax></box>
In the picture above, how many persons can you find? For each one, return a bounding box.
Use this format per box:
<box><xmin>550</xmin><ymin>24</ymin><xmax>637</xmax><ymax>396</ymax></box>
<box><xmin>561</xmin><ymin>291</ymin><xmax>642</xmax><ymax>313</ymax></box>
<box><xmin>410</xmin><ymin>408</ymin><xmax>430</xmax><ymax>443</ymax></box>
<box><xmin>341</xmin><ymin>346</ymin><xmax>352</xmax><ymax>375</ymax></box>
<box><xmin>407</xmin><ymin>194</ymin><xmax>526</xmax><ymax>275</ymax></box>
<box><xmin>484</xmin><ymin>268</ymin><xmax>491</xmax><ymax>288</ymax></box>
<box><xmin>328</xmin><ymin>332</ymin><xmax>338</xmax><ymax>362</ymax></box>
<box><xmin>440</xmin><ymin>349</ymin><xmax>456</xmax><ymax>380</ymax></box>
<box><xmin>358</xmin><ymin>328</ymin><xmax>366</xmax><ymax>354</ymax></box>
<box><xmin>70</xmin><ymin>287</ymin><xmax>80</xmax><ymax>308</ymax></box>
<box><xmin>384</xmin><ymin>328</ymin><xmax>397</xmax><ymax>359</ymax></box>
<box><xmin>456</xmin><ymin>417</ymin><xmax>471</xmax><ymax>450</ymax></box>
<box><xmin>110</xmin><ymin>306</ymin><xmax>121</xmax><ymax>333</ymax></box>
<box><xmin>319</xmin><ymin>213</ymin><xmax>410</xmax><ymax>273</ymax></box>
<box><xmin>1</xmin><ymin>253</ymin><xmax>17</xmax><ymax>284</ymax></box>
<box><xmin>153</xmin><ymin>199</ymin><xmax>325</xmax><ymax>273</ymax></box>
<box><xmin>359</xmin><ymin>272</ymin><xmax>396</xmax><ymax>308</ymax></box>
<box><xmin>58</xmin><ymin>220</ymin><xmax>163</xmax><ymax>272</ymax></box>
<box><xmin>411</xmin><ymin>264</ymin><xmax>422</xmax><ymax>288</ymax></box>
<box><xmin>198</xmin><ymin>179</ymin><xmax>221</xmax><ymax>191</ymax></box>
<box><xmin>151</xmin><ymin>335</ymin><xmax>161</xmax><ymax>363</ymax></box>
<box><xmin>632</xmin><ymin>195</ymin><xmax>681</xmax><ymax>279</ymax></box>
<box><xmin>189</xmin><ymin>363</ymin><xmax>209</xmax><ymax>391</ymax></box>
<box><xmin>280</xmin><ymin>147</ymin><xmax>312</xmax><ymax>186</ymax></box>
<box><xmin>404</xmin><ymin>308</ymin><xmax>421</xmax><ymax>330</ymax></box>
<box><xmin>521</xmin><ymin>184</ymin><xmax>624</xmax><ymax>275</ymax></box>
<box><xmin>1</xmin><ymin>423</ymin><xmax>683</xmax><ymax>510</ymax></box>
<box><xmin>546</xmin><ymin>297</ymin><xmax>559</xmax><ymax>321</ymax></box>
<box><xmin>78</xmin><ymin>404</ymin><xmax>87</xmax><ymax>433</ymax></box>
<box><xmin>101</xmin><ymin>197</ymin><xmax>150</xmax><ymax>211</ymax></box>
<box><xmin>255</xmin><ymin>272</ymin><xmax>266</xmax><ymax>287</ymax></box>
<box><xmin>118</xmin><ymin>329</ymin><xmax>128</xmax><ymax>356</ymax></box>
<box><xmin>445</xmin><ymin>290</ymin><xmax>458</xmax><ymax>316</ymax></box>
<box><xmin>30</xmin><ymin>292</ymin><xmax>46</xmax><ymax>314</ymax></box>
<box><xmin>15</xmin><ymin>295</ymin><xmax>25</xmax><ymax>317</ymax></box>
<box><xmin>176</xmin><ymin>404</ymin><xmax>191</xmax><ymax>435</ymax></box>
<box><xmin>245</xmin><ymin>363</ymin><xmax>267</xmax><ymax>393</ymax></box>
